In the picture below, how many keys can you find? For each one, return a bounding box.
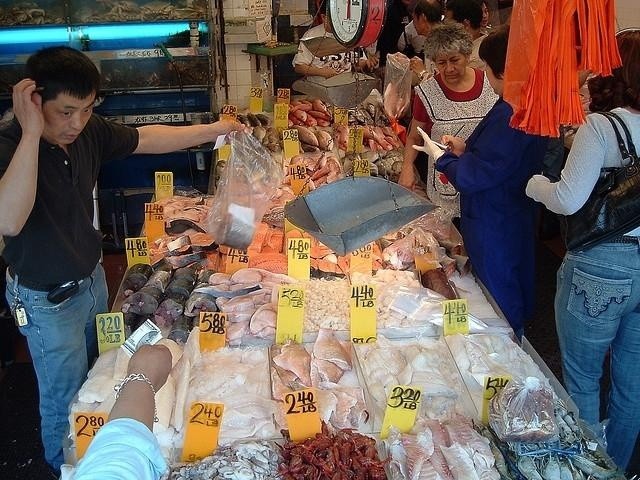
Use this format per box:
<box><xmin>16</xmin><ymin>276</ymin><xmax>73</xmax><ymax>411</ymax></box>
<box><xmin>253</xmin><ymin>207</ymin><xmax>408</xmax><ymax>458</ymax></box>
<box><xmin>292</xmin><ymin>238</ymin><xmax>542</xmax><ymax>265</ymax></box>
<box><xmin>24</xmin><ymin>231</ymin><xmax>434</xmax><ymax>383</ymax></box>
<box><xmin>10</xmin><ymin>298</ymin><xmax>30</xmax><ymax>329</ymax></box>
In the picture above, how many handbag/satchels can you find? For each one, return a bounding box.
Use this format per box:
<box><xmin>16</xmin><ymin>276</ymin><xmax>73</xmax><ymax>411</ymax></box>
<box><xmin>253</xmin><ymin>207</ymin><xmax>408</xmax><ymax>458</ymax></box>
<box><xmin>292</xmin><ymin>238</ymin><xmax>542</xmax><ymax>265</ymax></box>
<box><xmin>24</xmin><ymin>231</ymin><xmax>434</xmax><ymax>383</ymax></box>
<box><xmin>561</xmin><ymin>158</ymin><xmax>640</xmax><ymax>254</ymax></box>
<box><xmin>403</xmin><ymin>44</ymin><xmax>416</xmax><ymax>59</ymax></box>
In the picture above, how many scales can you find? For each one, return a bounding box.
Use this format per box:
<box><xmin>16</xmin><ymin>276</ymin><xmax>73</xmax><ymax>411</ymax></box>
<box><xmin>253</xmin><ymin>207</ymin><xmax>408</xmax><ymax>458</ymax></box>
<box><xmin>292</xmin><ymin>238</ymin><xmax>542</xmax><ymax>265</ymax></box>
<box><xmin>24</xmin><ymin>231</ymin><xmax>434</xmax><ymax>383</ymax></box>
<box><xmin>283</xmin><ymin>0</ymin><xmax>441</xmax><ymax>257</ymax></box>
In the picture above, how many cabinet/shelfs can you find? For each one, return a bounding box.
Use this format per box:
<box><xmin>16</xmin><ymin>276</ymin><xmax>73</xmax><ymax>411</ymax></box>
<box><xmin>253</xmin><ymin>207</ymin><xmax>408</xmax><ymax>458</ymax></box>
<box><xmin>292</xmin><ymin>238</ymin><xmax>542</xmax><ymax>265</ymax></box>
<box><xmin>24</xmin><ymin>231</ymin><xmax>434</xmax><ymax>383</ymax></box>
<box><xmin>1</xmin><ymin>0</ymin><xmax>215</xmax><ymax>120</ymax></box>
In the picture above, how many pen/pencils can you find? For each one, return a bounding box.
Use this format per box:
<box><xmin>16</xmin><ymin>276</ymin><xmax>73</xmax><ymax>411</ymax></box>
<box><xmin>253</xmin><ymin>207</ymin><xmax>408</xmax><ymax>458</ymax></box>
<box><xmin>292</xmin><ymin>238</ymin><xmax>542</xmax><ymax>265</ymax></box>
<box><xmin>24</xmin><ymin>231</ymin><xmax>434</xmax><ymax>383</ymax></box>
<box><xmin>444</xmin><ymin>124</ymin><xmax>465</xmax><ymax>146</ymax></box>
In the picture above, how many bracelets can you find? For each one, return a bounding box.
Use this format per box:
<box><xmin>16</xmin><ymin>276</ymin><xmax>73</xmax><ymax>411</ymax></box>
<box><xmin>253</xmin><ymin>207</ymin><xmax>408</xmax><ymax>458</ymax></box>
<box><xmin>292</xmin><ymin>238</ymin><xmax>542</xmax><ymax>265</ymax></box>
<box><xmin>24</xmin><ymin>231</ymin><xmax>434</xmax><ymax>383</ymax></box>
<box><xmin>113</xmin><ymin>373</ymin><xmax>159</xmax><ymax>422</ymax></box>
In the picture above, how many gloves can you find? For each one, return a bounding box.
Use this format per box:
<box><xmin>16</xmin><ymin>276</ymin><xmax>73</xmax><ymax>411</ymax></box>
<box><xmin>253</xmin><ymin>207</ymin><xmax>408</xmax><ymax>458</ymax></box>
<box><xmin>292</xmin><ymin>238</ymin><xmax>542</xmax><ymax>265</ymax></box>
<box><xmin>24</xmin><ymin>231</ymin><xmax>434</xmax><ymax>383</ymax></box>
<box><xmin>413</xmin><ymin>133</ymin><xmax>445</xmax><ymax>164</ymax></box>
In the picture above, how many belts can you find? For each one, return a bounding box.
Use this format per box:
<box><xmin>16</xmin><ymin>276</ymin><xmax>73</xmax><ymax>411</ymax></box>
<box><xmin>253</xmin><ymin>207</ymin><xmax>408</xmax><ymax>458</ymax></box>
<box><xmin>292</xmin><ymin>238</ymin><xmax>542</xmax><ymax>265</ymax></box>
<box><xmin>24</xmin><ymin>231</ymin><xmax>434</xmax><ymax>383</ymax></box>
<box><xmin>6</xmin><ymin>266</ymin><xmax>60</xmax><ymax>293</ymax></box>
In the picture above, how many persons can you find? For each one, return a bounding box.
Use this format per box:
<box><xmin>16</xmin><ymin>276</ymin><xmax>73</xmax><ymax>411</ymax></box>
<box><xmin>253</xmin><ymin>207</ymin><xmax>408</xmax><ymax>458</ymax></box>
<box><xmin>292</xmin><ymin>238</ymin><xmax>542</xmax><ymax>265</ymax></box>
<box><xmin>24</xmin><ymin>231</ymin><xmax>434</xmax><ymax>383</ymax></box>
<box><xmin>293</xmin><ymin>1</ymin><xmax>640</xmax><ymax>474</ymax></box>
<box><xmin>69</xmin><ymin>345</ymin><xmax>172</xmax><ymax>480</ymax></box>
<box><xmin>1</xmin><ymin>45</ymin><xmax>244</xmax><ymax>480</ymax></box>
